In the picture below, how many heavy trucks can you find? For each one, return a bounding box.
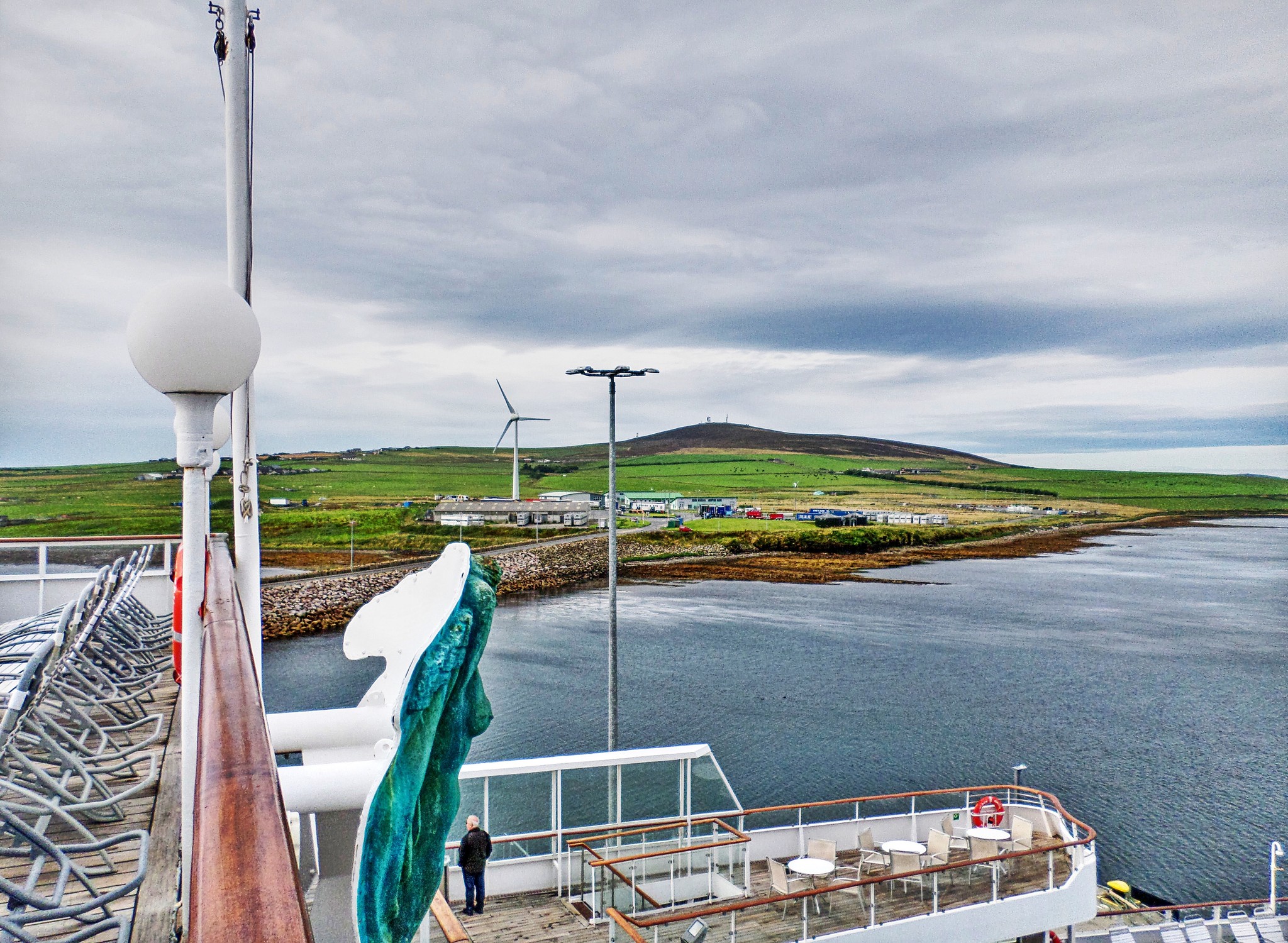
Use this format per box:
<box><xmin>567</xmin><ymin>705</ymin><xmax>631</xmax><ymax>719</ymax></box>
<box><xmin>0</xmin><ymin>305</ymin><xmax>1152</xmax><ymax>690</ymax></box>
<box><xmin>621</xmin><ymin>501</ymin><xmax>665</xmax><ymax>514</ymax></box>
<box><xmin>563</xmin><ymin>512</ymin><xmax>588</xmax><ymax>526</ymax></box>
<box><xmin>516</xmin><ymin>512</ymin><xmax>530</xmax><ymax>525</ymax></box>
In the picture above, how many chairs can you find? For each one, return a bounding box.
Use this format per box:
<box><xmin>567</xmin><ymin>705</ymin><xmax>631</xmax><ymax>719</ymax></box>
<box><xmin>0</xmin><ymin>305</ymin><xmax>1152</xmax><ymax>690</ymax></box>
<box><xmin>1159</xmin><ymin>915</ymin><xmax>1213</xmax><ymax>943</ymax></box>
<box><xmin>767</xmin><ymin>814</ymin><xmax>1035</xmax><ymax>920</ymax></box>
<box><xmin>0</xmin><ymin>543</ymin><xmax>175</xmax><ymax>943</ymax></box>
<box><xmin>1109</xmin><ymin>922</ymin><xmax>1137</xmax><ymax>943</ymax></box>
<box><xmin>1227</xmin><ymin>906</ymin><xmax>1288</xmax><ymax>943</ymax></box>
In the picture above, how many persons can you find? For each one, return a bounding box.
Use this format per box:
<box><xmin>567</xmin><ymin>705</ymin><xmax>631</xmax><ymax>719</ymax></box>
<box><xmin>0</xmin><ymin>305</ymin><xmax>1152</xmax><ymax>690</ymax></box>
<box><xmin>457</xmin><ymin>815</ymin><xmax>492</xmax><ymax>917</ymax></box>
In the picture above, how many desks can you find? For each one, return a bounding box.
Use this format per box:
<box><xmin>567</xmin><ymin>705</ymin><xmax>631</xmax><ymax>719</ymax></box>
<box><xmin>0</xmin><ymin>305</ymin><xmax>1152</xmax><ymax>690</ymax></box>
<box><xmin>882</xmin><ymin>839</ymin><xmax>927</xmax><ymax>893</ymax></box>
<box><xmin>788</xmin><ymin>858</ymin><xmax>835</xmax><ymax>916</ymax></box>
<box><xmin>966</xmin><ymin>827</ymin><xmax>1010</xmax><ymax>863</ymax></box>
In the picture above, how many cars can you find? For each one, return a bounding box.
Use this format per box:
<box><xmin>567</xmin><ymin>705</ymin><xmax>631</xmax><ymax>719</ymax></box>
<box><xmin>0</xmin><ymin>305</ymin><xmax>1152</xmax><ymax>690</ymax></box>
<box><xmin>679</xmin><ymin>525</ymin><xmax>692</xmax><ymax>532</ymax></box>
<box><xmin>813</xmin><ymin>491</ymin><xmax>825</xmax><ymax>495</ymax></box>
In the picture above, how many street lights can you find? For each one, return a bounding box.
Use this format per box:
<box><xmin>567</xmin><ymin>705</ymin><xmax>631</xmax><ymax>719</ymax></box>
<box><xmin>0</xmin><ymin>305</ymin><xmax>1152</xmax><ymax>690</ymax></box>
<box><xmin>132</xmin><ymin>268</ymin><xmax>261</xmax><ymax>943</ymax></box>
<box><xmin>566</xmin><ymin>366</ymin><xmax>661</xmax><ymax>848</ymax></box>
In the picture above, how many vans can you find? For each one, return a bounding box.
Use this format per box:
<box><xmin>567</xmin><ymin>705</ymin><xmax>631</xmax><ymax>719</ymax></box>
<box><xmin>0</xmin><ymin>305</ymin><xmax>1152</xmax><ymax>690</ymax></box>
<box><xmin>769</xmin><ymin>513</ymin><xmax>795</xmax><ymax>521</ymax></box>
<box><xmin>737</xmin><ymin>507</ymin><xmax>762</xmax><ymax>518</ymax></box>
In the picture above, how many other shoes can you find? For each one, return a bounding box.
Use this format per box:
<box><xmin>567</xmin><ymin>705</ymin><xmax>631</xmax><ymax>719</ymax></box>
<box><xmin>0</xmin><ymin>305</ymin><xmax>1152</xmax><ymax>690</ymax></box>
<box><xmin>463</xmin><ymin>908</ymin><xmax>474</xmax><ymax>916</ymax></box>
<box><xmin>473</xmin><ymin>906</ymin><xmax>483</xmax><ymax>914</ymax></box>
